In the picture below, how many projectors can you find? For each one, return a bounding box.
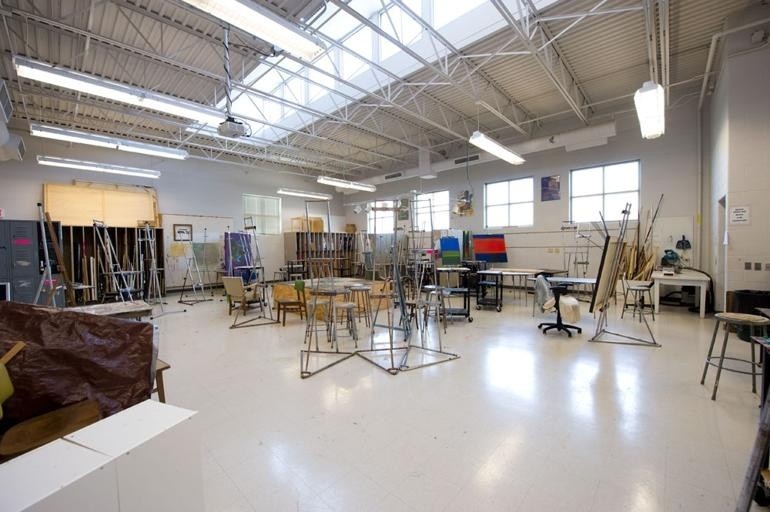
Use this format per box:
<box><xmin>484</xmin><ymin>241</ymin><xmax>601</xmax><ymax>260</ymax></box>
<box><xmin>217</xmin><ymin>121</ymin><xmax>245</xmax><ymax>138</ymax></box>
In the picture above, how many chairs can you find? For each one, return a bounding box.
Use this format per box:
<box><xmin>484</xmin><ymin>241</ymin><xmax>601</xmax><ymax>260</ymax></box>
<box><xmin>275</xmin><ymin>279</ymin><xmax>307</xmax><ymax>326</ymax></box>
<box><xmin>534</xmin><ymin>272</ymin><xmax>582</xmax><ymax>337</ymax></box>
<box><xmin>223</xmin><ymin>276</ymin><xmax>267</xmax><ymax>315</ymax></box>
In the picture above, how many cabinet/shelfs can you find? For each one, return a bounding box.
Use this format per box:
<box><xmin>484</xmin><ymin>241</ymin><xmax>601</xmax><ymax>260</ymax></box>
<box><xmin>1</xmin><ymin>219</ymin><xmax>65</xmax><ymax>308</ymax></box>
<box><xmin>285</xmin><ymin>232</ymin><xmax>356</xmax><ymax>276</ymax></box>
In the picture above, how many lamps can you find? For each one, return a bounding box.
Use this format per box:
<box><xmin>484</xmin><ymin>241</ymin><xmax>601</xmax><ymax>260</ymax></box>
<box><xmin>33</xmin><ymin>152</ymin><xmax>163</xmax><ymax>180</ymax></box>
<box><xmin>28</xmin><ymin>123</ymin><xmax>191</xmax><ymax>162</ymax></box>
<box><xmin>633</xmin><ymin>81</ymin><xmax>667</xmax><ymax>141</ymax></box>
<box><xmin>274</xmin><ymin>182</ymin><xmax>332</xmax><ymax>202</ymax></box>
<box><xmin>316</xmin><ymin>175</ymin><xmax>377</xmax><ymax>198</ymax></box>
<box><xmin>469</xmin><ymin>99</ymin><xmax>525</xmax><ymax>166</ymax></box>
<box><xmin>9</xmin><ymin>54</ymin><xmax>234</xmax><ymax>129</ymax></box>
<box><xmin>451</xmin><ymin>200</ymin><xmax>462</xmax><ymax>216</ymax></box>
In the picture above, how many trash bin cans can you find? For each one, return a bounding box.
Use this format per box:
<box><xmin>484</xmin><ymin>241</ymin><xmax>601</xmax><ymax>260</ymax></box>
<box><xmin>734</xmin><ymin>289</ymin><xmax>770</xmax><ymax>344</ymax></box>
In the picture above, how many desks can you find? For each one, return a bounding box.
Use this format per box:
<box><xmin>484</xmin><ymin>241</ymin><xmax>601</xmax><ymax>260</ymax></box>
<box><xmin>475</xmin><ymin>266</ymin><xmax>596</xmax><ymax>316</ymax></box>
<box><xmin>151</xmin><ymin>356</ymin><xmax>171</xmax><ymax>403</ymax></box>
<box><xmin>651</xmin><ymin>269</ymin><xmax>711</xmax><ymax>318</ymax></box>
<box><xmin>1</xmin><ymin>392</ymin><xmax>102</xmax><ymax>460</ymax></box>
<box><xmin>35</xmin><ymin>298</ymin><xmax>153</xmax><ymax>323</ymax></box>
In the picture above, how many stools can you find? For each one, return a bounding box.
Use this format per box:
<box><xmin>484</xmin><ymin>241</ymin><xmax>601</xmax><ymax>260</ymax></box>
<box><xmin>699</xmin><ymin>309</ymin><xmax>770</xmax><ymax>401</ymax></box>
<box><xmin>621</xmin><ymin>286</ymin><xmax>654</xmax><ymax>323</ymax></box>
<box><xmin>303</xmin><ymin>275</ymin><xmax>471</xmax><ymax>353</ymax></box>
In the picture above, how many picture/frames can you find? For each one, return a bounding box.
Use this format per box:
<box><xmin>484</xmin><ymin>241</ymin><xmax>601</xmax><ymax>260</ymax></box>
<box><xmin>173</xmin><ymin>223</ymin><xmax>193</xmax><ymax>241</ymax></box>
